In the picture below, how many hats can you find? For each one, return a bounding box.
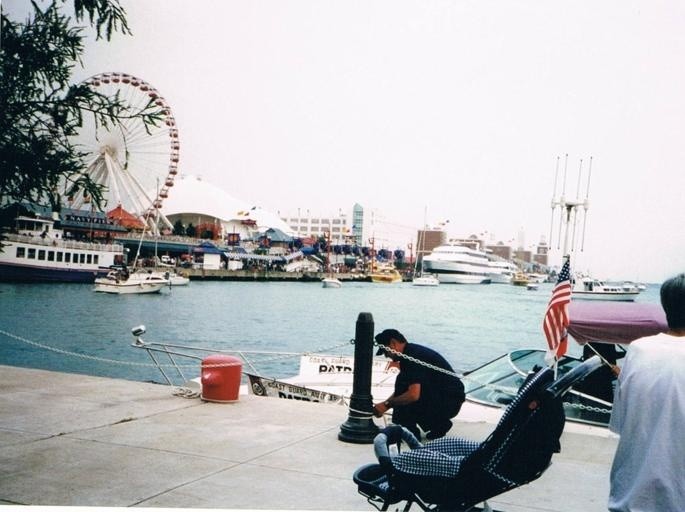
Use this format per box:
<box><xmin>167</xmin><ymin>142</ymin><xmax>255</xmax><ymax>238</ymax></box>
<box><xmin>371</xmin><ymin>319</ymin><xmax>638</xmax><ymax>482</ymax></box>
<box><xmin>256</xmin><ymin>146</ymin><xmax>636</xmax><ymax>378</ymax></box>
<box><xmin>375</xmin><ymin>330</ymin><xmax>400</xmax><ymax>355</ymax></box>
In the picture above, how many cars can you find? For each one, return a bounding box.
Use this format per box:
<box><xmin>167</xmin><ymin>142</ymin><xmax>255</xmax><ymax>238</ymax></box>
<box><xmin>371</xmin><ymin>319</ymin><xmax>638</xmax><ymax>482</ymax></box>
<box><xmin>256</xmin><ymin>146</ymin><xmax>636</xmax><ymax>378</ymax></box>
<box><xmin>160</xmin><ymin>256</ymin><xmax>170</xmax><ymax>263</ymax></box>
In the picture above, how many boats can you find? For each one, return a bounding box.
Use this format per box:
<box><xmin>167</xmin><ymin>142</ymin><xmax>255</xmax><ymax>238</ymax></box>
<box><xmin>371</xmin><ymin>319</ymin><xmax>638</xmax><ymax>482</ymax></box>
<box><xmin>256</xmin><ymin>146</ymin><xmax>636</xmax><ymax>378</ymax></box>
<box><xmin>129</xmin><ymin>302</ymin><xmax>670</xmax><ymax>439</ymax></box>
<box><xmin>0</xmin><ymin>212</ymin><xmax>130</xmax><ymax>284</ymax></box>
<box><xmin>92</xmin><ymin>261</ymin><xmax>170</xmax><ymax>294</ymax></box>
<box><xmin>571</xmin><ymin>269</ymin><xmax>646</xmax><ymax>301</ymax></box>
<box><xmin>322</xmin><ymin>238</ymin><xmax>562</xmax><ymax>291</ymax></box>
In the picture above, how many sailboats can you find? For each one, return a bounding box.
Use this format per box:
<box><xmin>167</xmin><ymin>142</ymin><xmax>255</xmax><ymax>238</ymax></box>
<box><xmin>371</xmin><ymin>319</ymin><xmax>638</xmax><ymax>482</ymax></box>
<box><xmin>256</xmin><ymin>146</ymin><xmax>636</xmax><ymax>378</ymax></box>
<box><xmin>130</xmin><ymin>176</ymin><xmax>190</xmax><ymax>288</ymax></box>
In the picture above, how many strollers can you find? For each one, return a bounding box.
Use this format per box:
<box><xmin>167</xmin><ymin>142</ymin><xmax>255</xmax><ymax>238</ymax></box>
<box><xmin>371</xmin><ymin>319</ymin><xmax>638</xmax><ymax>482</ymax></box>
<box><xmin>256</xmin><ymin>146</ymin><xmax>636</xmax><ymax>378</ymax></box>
<box><xmin>352</xmin><ymin>351</ymin><xmax>604</xmax><ymax>511</ymax></box>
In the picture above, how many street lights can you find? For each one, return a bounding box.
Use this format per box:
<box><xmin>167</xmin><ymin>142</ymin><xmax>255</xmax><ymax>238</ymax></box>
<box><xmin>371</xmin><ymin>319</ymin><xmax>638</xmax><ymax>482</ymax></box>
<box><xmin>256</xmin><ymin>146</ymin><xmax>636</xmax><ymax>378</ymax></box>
<box><xmin>324</xmin><ymin>230</ymin><xmax>330</xmax><ymax>274</ymax></box>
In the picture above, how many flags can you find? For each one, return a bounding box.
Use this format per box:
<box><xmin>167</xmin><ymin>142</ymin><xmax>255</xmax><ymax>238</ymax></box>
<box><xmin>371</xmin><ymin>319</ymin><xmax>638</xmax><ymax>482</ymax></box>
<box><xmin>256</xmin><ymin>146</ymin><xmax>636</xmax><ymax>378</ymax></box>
<box><xmin>542</xmin><ymin>256</ymin><xmax>575</xmax><ymax>368</ymax></box>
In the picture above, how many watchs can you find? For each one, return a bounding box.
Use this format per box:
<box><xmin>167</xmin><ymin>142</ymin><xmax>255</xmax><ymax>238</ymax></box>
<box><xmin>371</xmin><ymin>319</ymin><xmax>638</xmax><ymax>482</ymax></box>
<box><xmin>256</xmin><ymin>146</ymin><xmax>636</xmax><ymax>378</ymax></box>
<box><xmin>384</xmin><ymin>399</ymin><xmax>393</xmax><ymax>409</ymax></box>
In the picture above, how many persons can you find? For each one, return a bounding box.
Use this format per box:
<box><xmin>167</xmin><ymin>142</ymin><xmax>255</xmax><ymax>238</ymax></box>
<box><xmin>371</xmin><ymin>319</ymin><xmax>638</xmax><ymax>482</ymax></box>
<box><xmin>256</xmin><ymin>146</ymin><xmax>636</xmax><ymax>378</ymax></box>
<box><xmin>580</xmin><ymin>342</ymin><xmax>620</xmax><ymax>424</ymax></box>
<box><xmin>607</xmin><ymin>272</ymin><xmax>685</xmax><ymax>512</ymax></box>
<box><xmin>372</xmin><ymin>329</ymin><xmax>465</xmax><ymax>443</ymax></box>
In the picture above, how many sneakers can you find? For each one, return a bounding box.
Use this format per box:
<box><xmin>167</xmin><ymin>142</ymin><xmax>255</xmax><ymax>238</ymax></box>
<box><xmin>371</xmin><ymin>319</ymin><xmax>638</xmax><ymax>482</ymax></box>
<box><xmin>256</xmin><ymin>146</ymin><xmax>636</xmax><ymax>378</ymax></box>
<box><xmin>426</xmin><ymin>420</ymin><xmax>453</xmax><ymax>440</ymax></box>
<box><xmin>412</xmin><ymin>433</ymin><xmax>421</xmax><ymax>442</ymax></box>
